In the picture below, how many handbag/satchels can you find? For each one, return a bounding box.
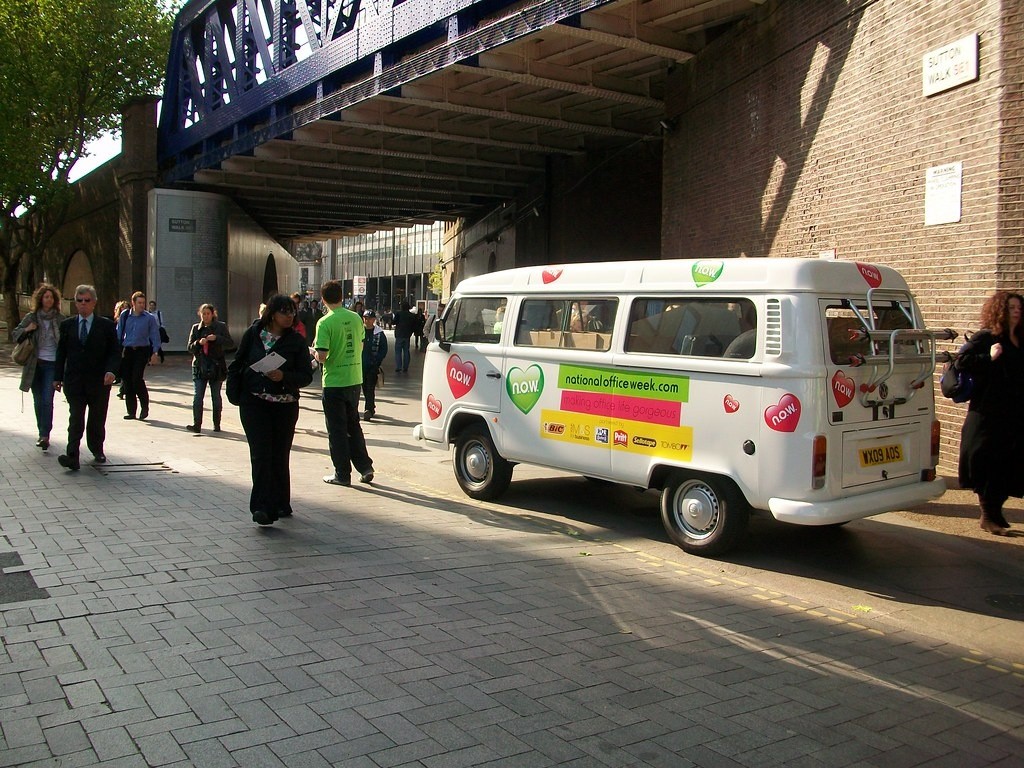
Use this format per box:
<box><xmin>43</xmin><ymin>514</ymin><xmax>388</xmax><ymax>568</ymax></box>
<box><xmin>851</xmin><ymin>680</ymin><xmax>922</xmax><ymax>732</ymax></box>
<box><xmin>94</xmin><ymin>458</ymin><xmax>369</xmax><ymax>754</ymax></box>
<box><xmin>226</xmin><ymin>360</ymin><xmax>255</xmax><ymax>406</ymax></box>
<box><xmin>941</xmin><ymin>359</ymin><xmax>976</xmax><ymax>405</ymax></box>
<box><xmin>11</xmin><ymin>336</ymin><xmax>33</xmax><ymax>366</ymax></box>
<box><xmin>377</xmin><ymin>368</ymin><xmax>384</xmax><ymax>388</ymax></box>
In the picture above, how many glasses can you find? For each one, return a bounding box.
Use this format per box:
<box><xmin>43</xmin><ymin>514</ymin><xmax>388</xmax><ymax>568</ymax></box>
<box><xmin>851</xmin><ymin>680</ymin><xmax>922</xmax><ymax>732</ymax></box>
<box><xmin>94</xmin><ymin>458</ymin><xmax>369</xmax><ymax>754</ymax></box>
<box><xmin>76</xmin><ymin>298</ymin><xmax>90</xmax><ymax>302</ymax></box>
<box><xmin>279</xmin><ymin>307</ymin><xmax>294</xmax><ymax>315</ymax></box>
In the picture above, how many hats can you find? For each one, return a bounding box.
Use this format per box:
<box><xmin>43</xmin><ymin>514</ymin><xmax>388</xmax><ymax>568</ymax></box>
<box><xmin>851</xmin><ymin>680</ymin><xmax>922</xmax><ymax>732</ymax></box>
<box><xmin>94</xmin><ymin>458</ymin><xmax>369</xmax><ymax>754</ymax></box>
<box><xmin>362</xmin><ymin>308</ymin><xmax>376</xmax><ymax>318</ymax></box>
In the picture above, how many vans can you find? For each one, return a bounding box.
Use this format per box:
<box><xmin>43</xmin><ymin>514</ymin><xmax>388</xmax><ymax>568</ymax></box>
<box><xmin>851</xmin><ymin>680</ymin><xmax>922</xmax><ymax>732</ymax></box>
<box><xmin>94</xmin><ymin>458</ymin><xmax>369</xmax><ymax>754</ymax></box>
<box><xmin>414</xmin><ymin>256</ymin><xmax>945</xmax><ymax>556</ymax></box>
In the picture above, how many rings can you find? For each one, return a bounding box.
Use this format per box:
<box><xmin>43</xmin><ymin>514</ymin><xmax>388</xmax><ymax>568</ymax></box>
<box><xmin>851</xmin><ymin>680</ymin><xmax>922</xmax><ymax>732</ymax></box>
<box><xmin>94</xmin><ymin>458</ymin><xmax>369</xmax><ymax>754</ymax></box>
<box><xmin>273</xmin><ymin>377</ymin><xmax>276</xmax><ymax>379</ymax></box>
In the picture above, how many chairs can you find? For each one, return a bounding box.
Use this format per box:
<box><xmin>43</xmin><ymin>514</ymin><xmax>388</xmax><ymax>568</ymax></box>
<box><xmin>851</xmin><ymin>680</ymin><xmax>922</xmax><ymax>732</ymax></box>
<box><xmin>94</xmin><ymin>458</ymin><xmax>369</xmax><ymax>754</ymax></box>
<box><xmin>723</xmin><ymin>329</ymin><xmax>755</xmax><ymax>358</ymax></box>
<box><xmin>526</xmin><ymin>304</ymin><xmax>559</xmax><ymax>331</ymax></box>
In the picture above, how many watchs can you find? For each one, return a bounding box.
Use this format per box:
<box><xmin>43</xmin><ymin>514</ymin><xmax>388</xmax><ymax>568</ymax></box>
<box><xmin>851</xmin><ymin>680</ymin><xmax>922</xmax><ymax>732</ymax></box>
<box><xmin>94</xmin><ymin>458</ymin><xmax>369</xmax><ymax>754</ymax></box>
<box><xmin>153</xmin><ymin>352</ymin><xmax>158</xmax><ymax>355</ymax></box>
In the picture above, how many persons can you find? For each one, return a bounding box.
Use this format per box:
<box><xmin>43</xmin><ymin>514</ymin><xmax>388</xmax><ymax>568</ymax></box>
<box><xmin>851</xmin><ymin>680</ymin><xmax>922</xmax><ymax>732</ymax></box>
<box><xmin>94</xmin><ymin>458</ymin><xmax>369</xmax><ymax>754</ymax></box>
<box><xmin>955</xmin><ymin>291</ymin><xmax>1024</xmax><ymax>535</ymax></box>
<box><xmin>493</xmin><ymin>306</ymin><xmax>506</xmax><ymax>335</ymax></box>
<box><xmin>146</xmin><ymin>301</ymin><xmax>166</xmax><ymax>366</ymax></box>
<box><xmin>361</xmin><ymin>309</ymin><xmax>388</xmax><ymax>422</ymax></box>
<box><xmin>286</xmin><ymin>291</ymin><xmax>446</xmax><ymax>374</ymax></box>
<box><xmin>310</xmin><ymin>282</ymin><xmax>375</xmax><ymax>487</ymax></box>
<box><xmin>116</xmin><ymin>291</ymin><xmax>161</xmax><ymax>419</ymax></box>
<box><xmin>226</xmin><ymin>294</ymin><xmax>313</xmax><ymax>525</ymax></box>
<box><xmin>112</xmin><ymin>300</ymin><xmax>132</xmax><ymax>385</ymax></box>
<box><xmin>52</xmin><ymin>284</ymin><xmax>120</xmax><ymax>470</ymax></box>
<box><xmin>12</xmin><ymin>284</ymin><xmax>66</xmax><ymax>450</ymax></box>
<box><xmin>567</xmin><ymin>310</ymin><xmax>590</xmax><ymax>333</ymax></box>
<box><xmin>187</xmin><ymin>303</ymin><xmax>229</xmax><ymax>433</ymax></box>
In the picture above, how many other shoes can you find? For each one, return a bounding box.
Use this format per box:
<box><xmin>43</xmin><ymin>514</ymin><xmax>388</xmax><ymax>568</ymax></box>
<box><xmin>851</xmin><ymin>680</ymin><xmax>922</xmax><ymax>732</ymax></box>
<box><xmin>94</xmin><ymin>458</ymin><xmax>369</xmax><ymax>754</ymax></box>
<box><xmin>124</xmin><ymin>415</ymin><xmax>136</xmax><ymax>419</ymax></box>
<box><xmin>279</xmin><ymin>506</ymin><xmax>293</xmax><ymax>516</ymax></box>
<box><xmin>322</xmin><ymin>476</ymin><xmax>351</xmax><ymax>486</ymax></box>
<box><xmin>40</xmin><ymin>438</ymin><xmax>50</xmax><ymax>450</ymax></box>
<box><xmin>160</xmin><ymin>355</ymin><xmax>165</xmax><ymax>364</ymax></box>
<box><xmin>139</xmin><ymin>411</ymin><xmax>148</xmax><ymax>420</ymax></box>
<box><xmin>252</xmin><ymin>510</ymin><xmax>279</xmax><ymax>525</ymax></box>
<box><xmin>359</xmin><ymin>469</ymin><xmax>374</xmax><ymax>482</ymax></box>
<box><xmin>36</xmin><ymin>436</ymin><xmax>43</xmax><ymax>446</ymax></box>
<box><xmin>58</xmin><ymin>454</ymin><xmax>80</xmax><ymax>470</ymax></box>
<box><xmin>213</xmin><ymin>423</ymin><xmax>221</xmax><ymax>431</ymax></box>
<box><xmin>364</xmin><ymin>409</ymin><xmax>371</xmax><ymax>421</ymax></box>
<box><xmin>186</xmin><ymin>425</ymin><xmax>202</xmax><ymax>433</ymax></box>
<box><xmin>94</xmin><ymin>453</ymin><xmax>106</xmax><ymax>462</ymax></box>
<box><xmin>117</xmin><ymin>393</ymin><xmax>123</xmax><ymax>399</ymax></box>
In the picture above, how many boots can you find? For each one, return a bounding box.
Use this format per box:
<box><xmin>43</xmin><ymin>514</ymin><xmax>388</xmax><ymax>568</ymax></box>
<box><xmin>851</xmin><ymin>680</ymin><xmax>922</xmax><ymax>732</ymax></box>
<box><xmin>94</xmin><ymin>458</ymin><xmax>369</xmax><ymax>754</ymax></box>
<box><xmin>978</xmin><ymin>496</ymin><xmax>1010</xmax><ymax>536</ymax></box>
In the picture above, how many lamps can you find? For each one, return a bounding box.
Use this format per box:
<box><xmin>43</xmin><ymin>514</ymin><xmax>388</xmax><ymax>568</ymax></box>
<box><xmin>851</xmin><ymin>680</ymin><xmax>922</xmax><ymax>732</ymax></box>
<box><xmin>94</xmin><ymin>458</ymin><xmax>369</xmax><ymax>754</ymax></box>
<box><xmin>658</xmin><ymin>116</ymin><xmax>680</xmax><ymax>132</ymax></box>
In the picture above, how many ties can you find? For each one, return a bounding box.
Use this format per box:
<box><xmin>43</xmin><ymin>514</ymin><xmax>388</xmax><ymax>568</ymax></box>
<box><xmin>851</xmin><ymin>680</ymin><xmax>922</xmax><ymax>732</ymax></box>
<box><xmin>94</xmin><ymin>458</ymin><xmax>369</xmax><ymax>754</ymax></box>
<box><xmin>79</xmin><ymin>320</ymin><xmax>87</xmax><ymax>347</ymax></box>
